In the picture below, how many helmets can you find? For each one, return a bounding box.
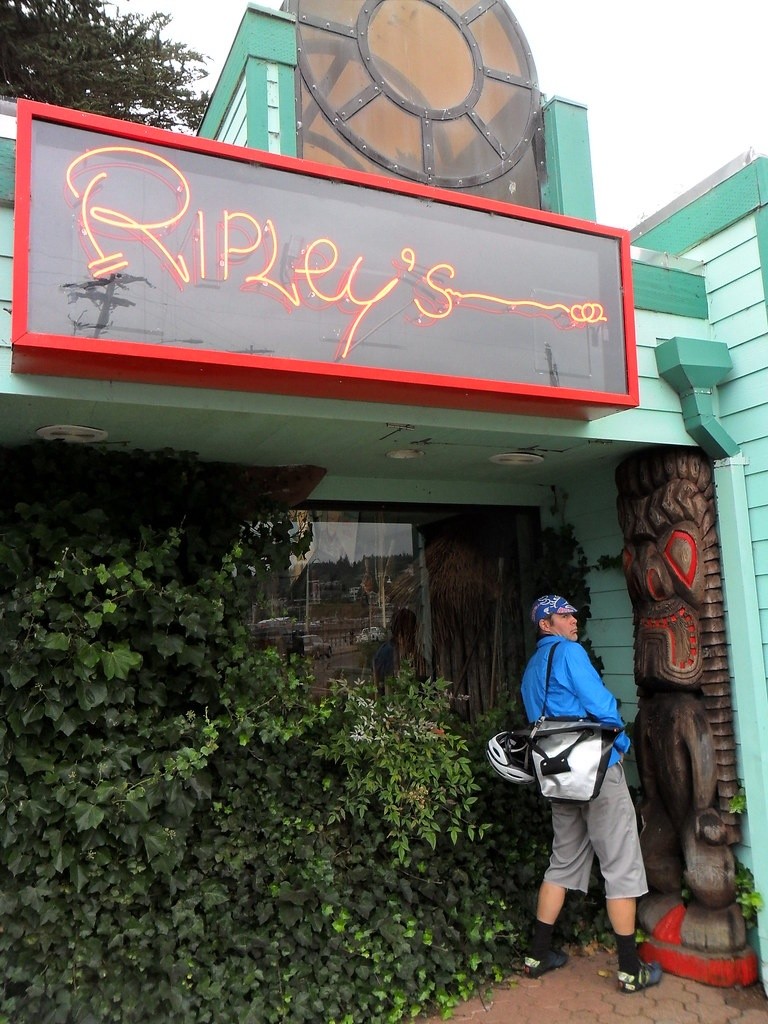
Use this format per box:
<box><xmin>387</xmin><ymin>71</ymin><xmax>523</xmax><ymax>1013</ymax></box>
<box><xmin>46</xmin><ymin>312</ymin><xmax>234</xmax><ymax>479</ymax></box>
<box><xmin>485</xmin><ymin>731</ymin><xmax>535</xmax><ymax>784</ymax></box>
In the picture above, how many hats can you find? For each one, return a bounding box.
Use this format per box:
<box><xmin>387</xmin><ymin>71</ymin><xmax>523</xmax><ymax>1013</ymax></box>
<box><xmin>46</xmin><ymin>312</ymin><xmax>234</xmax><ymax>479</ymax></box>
<box><xmin>529</xmin><ymin>595</ymin><xmax>578</xmax><ymax>633</ymax></box>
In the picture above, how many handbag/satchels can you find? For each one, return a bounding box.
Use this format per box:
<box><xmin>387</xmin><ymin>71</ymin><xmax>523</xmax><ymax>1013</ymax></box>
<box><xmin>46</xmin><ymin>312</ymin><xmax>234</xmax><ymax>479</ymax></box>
<box><xmin>532</xmin><ymin>717</ymin><xmax>626</xmax><ymax>804</ymax></box>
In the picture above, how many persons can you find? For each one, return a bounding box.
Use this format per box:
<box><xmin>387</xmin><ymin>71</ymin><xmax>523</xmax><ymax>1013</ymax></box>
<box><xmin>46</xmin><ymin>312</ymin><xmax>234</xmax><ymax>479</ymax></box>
<box><xmin>374</xmin><ymin>608</ymin><xmax>425</xmax><ymax>695</ymax></box>
<box><xmin>521</xmin><ymin>596</ymin><xmax>663</xmax><ymax>994</ymax></box>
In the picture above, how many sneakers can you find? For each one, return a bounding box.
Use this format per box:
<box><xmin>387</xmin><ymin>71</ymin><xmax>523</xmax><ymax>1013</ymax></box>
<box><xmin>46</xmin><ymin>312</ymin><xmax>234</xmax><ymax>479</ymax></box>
<box><xmin>525</xmin><ymin>944</ymin><xmax>568</xmax><ymax>979</ymax></box>
<box><xmin>617</xmin><ymin>957</ymin><xmax>662</xmax><ymax>993</ymax></box>
